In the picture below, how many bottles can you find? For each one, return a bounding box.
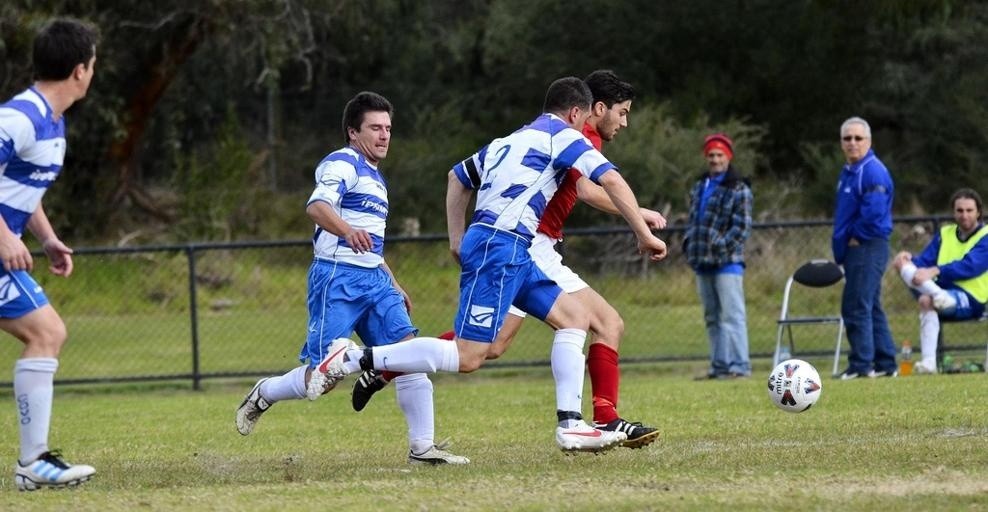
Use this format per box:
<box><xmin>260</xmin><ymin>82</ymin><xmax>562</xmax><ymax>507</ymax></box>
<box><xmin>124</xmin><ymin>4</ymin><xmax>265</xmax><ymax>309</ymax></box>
<box><xmin>900</xmin><ymin>341</ymin><xmax>913</xmax><ymax>376</ymax></box>
<box><xmin>779</xmin><ymin>346</ymin><xmax>791</xmax><ymax>363</ymax></box>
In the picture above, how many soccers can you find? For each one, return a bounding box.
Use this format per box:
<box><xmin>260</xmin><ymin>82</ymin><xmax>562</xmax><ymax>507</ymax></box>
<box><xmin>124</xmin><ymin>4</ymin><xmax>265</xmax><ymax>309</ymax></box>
<box><xmin>768</xmin><ymin>359</ymin><xmax>822</xmax><ymax>414</ymax></box>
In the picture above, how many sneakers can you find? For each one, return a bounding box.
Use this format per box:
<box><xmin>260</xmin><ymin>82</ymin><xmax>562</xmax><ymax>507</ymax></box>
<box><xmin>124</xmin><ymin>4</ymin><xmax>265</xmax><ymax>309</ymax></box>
<box><xmin>831</xmin><ymin>367</ymin><xmax>875</xmax><ymax>380</ymax></box>
<box><xmin>407</xmin><ymin>435</ymin><xmax>470</xmax><ymax>466</ymax></box>
<box><xmin>590</xmin><ymin>417</ymin><xmax>660</xmax><ymax>455</ymax></box>
<box><xmin>915</xmin><ymin>359</ymin><xmax>938</xmax><ymax>374</ymax></box>
<box><xmin>14</xmin><ymin>448</ymin><xmax>98</xmax><ymax>492</ymax></box>
<box><xmin>728</xmin><ymin>362</ymin><xmax>751</xmax><ymax>376</ymax></box>
<box><xmin>933</xmin><ymin>289</ymin><xmax>957</xmax><ymax>311</ymax></box>
<box><xmin>235</xmin><ymin>376</ymin><xmax>271</xmax><ymax>433</ymax></box>
<box><xmin>306</xmin><ymin>337</ymin><xmax>359</xmax><ymax>401</ymax></box>
<box><xmin>351</xmin><ymin>368</ymin><xmax>388</xmax><ymax>411</ymax></box>
<box><xmin>554</xmin><ymin>418</ymin><xmax>628</xmax><ymax>456</ymax></box>
<box><xmin>708</xmin><ymin>367</ymin><xmax>728</xmax><ymax>376</ymax></box>
<box><xmin>873</xmin><ymin>368</ymin><xmax>899</xmax><ymax>378</ymax></box>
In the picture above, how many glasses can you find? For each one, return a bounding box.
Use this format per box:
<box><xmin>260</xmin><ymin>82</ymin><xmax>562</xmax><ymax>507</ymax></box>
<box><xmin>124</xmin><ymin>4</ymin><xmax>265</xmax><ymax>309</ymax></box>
<box><xmin>843</xmin><ymin>136</ymin><xmax>868</xmax><ymax>141</ymax></box>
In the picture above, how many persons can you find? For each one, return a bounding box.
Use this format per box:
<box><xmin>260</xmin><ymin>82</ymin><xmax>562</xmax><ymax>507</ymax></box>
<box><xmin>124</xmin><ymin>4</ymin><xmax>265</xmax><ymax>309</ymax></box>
<box><xmin>678</xmin><ymin>132</ymin><xmax>752</xmax><ymax>381</ymax></box>
<box><xmin>894</xmin><ymin>186</ymin><xmax>988</xmax><ymax>377</ymax></box>
<box><xmin>0</xmin><ymin>20</ymin><xmax>102</xmax><ymax>493</ymax></box>
<box><xmin>830</xmin><ymin>116</ymin><xmax>899</xmax><ymax>380</ymax></box>
<box><xmin>235</xmin><ymin>92</ymin><xmax>470</xmax><ymax>465</ymax></box>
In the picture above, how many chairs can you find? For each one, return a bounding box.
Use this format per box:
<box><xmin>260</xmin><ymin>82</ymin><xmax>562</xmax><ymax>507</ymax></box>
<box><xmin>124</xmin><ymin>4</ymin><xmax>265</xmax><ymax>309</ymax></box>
<box><xmin>937</xmin><ymin>310</ymin><xmax>988</xmax><ymax>374</ymax></box>
<box><xmin>774</xmin><ymin>260</ymin><xmax>846</xmax><ymax>376</ymax></box>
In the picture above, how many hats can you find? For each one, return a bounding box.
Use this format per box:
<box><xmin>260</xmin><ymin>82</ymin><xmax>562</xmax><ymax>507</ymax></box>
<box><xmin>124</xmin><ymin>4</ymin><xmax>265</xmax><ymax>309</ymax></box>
<box><xmin>703</xmin><ymin>133</ymin><xmax>736</xmax><ymax>160</ymax></box>
